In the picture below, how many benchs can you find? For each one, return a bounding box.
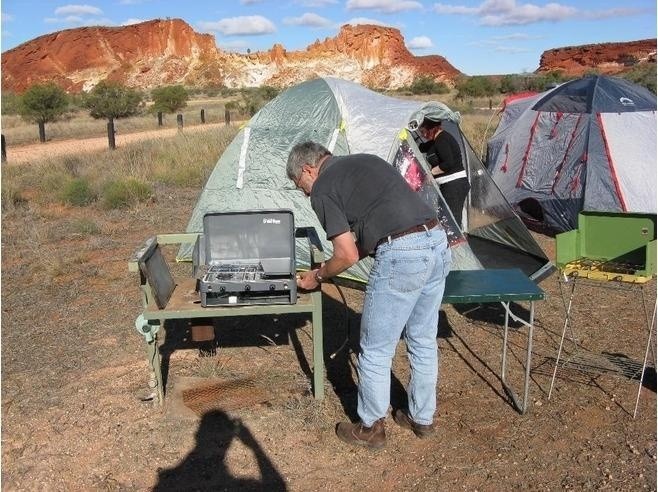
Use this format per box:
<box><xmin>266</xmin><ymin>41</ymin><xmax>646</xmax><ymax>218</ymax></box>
<box><xmin>128</xmin><ymin>225</ymin><xmax>325</xmax><ymax>404</ymax></box>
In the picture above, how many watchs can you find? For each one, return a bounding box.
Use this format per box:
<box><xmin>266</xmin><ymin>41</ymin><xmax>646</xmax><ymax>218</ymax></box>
<box><xmin>314</xmin><ymin>272</ymin><xmax>326</xmax><ymax>283</ymax></box>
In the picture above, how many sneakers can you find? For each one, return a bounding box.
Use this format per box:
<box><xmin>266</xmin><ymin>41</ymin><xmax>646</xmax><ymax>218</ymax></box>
<box><xmin>336</xmin><ymin>418</ymin><xmax>387</xmax><ymax>449</ymax></box>
<box><xmin>394</xmin><ymin>408</ymin><xmax>434</xmax><ymax>438</ymax></box>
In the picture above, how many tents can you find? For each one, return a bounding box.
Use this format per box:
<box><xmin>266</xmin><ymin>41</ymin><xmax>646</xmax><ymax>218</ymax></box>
<box><xmin>176</xmin><ymin>76</ymin><xmax>557</xmax><ymax>286</ymax></box>
<box><xmin>471</xmin><ymin>74</ymin><xmax>657</xmax><ymax>238</ymax></box>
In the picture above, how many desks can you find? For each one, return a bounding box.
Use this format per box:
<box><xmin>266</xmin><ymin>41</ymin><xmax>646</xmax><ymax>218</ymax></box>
<box><xmin>441</xmin><ymin>268</ymin><xmax>542</xmax><ymax>416</ymax></box>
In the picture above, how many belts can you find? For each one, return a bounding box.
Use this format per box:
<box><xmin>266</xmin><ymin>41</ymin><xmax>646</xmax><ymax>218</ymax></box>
<box><xmin>377</xmin><ymin>217</ymin><xmax>439</xmax><ymax>246</ymax></box>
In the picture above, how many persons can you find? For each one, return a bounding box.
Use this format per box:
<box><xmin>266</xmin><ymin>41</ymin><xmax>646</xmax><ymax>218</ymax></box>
<box><xmin>419</xmin><ymin>117</ymin><xmax>471</xmax><ymax>248</ymax></box>
<box><xmin>286</xmin><ymin>141</ymin><xmax>452</xmax><ymax>449</ymax></box>
